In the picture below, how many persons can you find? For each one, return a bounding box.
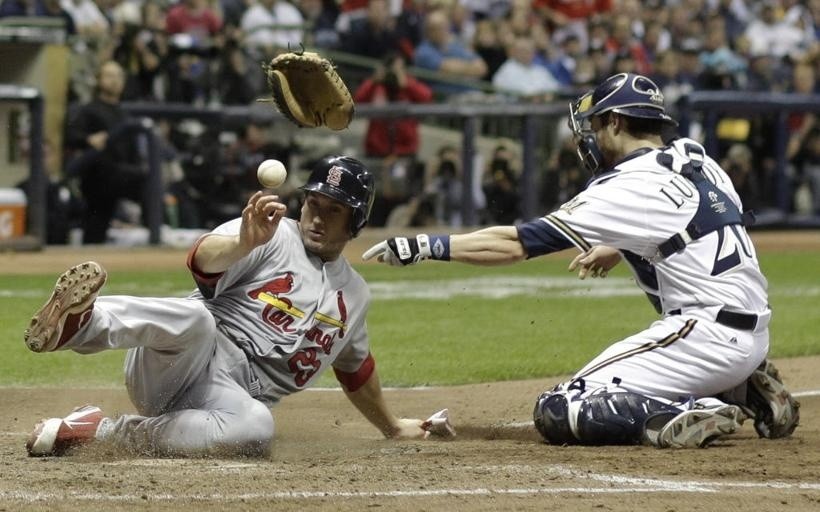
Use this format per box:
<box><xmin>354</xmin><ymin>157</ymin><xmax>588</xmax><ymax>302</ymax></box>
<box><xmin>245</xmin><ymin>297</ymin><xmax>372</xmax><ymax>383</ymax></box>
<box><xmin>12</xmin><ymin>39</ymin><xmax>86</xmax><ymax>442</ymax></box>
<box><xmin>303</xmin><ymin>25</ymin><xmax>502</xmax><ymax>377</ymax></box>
<box><xmin>24</xmin><ymin>156</ymin><xmax>460</xmax><ymax>461</ymax></box>
<box><xmin>360</xmin><ymin>74</ymin><xmax>800</xmax><ymax>448</ymax></box>
<box><xmin>1</xmin><ymin>0</ymin><xmax>820</xmax><ymax>251</ymax></box>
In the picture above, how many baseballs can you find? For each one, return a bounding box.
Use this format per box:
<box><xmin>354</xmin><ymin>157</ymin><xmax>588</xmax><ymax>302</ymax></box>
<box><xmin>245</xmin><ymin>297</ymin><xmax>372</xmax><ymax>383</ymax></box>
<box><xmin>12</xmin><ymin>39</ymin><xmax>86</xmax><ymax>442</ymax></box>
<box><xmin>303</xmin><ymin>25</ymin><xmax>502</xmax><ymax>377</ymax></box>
<box><xmin>256</xmin><ymin>159</ymin><xmax>287</xmax><ymax>189</ymax></box>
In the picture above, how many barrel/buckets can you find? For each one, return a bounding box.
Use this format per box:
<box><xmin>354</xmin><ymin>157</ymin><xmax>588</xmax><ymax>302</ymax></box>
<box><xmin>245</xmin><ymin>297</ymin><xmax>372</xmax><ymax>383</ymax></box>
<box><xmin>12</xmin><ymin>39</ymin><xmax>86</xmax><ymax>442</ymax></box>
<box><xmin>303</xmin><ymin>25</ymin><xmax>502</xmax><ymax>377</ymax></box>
<box><xmin>0</xmin><ymin>188</ymin><xmax>30</xmax><ymax>240</ymax></box>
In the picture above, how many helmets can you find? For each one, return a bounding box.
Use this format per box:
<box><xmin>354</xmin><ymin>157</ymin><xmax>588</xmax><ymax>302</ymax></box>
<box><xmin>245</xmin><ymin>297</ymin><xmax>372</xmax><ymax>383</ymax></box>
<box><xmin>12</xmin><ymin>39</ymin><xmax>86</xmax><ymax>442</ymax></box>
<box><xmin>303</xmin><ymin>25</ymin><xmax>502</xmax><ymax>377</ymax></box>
<box><xmin>588</xmin><ymin>71</ymin><xmax>680</xmax><ymax>128</ymax></box>
<box><xmin>297</xmin><ymin>152</ymin><xmax>376</xmax><ymax>240</ymax></box>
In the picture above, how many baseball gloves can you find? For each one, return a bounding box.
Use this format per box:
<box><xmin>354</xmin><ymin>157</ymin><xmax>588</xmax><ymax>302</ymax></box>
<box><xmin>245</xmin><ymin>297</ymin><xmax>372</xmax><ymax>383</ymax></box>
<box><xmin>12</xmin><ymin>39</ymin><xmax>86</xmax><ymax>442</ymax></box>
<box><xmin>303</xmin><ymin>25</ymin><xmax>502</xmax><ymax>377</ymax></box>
<box><xmin>256</xmin><ymin>42</ymin><xmax>355</xmax><ymax>131</ymax></box>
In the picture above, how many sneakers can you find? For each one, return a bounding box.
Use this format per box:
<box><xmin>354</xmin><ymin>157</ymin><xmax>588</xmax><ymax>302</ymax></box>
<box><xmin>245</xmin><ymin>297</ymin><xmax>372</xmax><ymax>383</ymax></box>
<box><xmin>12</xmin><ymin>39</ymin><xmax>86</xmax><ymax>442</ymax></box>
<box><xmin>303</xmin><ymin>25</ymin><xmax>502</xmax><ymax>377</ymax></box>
<box><xmin>23</xmin><ymin>260</ymin><xmax>108</xmax><ymax>354</ymax></box>
<box><xmin>656</xmin><ymin>396</ymin><xmax>744</xmax><ymax>450</ymax></box>
<box><xmin>747</xmin><ymin>357</ymin><xmax>801</xmax><ymax>438</ymax></box>
<box><xmin>25</xmin><ymin>405</ymin><xmax>116</xmax><ymax>457</ymax></box>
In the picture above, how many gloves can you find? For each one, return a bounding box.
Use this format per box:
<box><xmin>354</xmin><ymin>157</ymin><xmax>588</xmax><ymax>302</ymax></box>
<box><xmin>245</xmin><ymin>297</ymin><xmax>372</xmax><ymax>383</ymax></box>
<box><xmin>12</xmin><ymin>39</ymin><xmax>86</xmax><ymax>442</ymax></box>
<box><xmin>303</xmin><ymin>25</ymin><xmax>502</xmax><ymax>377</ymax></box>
<box><xmin>361</xmin><ymin>233</ymin><xmax>433</xmax><ymax>267</ymax></box>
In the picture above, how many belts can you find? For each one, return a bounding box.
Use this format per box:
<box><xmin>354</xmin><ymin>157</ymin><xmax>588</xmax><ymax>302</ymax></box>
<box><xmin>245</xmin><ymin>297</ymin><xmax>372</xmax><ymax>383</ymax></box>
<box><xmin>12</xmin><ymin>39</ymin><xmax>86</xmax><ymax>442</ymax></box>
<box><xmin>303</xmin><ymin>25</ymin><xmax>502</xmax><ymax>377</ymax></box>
<box><xmin>667</xmin><ymin>308</ymin><xmax>758</xmax><ymax>329</ymax></box>
